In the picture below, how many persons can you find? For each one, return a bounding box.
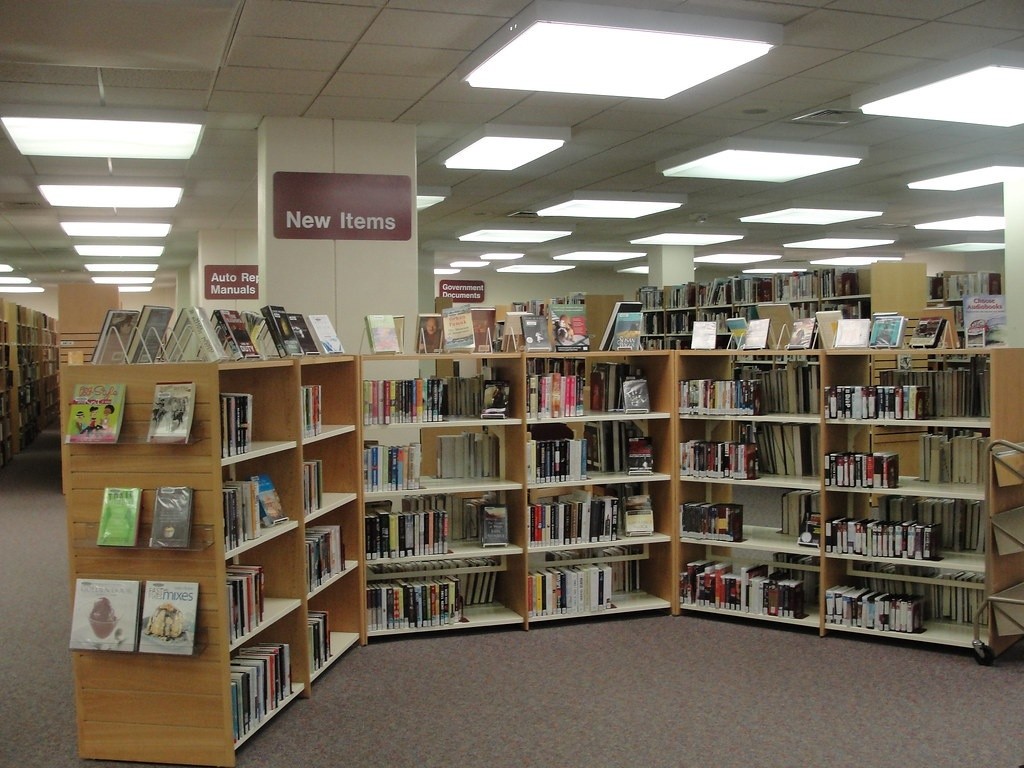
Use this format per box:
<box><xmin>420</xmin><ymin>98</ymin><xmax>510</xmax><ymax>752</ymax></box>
<box><xmin>418</xmin><ymin>318</ymin><xmax>442</xmax><ymax>353</ymax></box>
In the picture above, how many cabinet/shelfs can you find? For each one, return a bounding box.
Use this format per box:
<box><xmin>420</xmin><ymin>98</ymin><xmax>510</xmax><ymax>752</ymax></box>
<box><xmin>0</xmin><ymin>297</ymin><xmax>58</xmax><ymax>469</ymax></box>
<box><xmin>927</xmin><ymin>271</ymin><xmax>1005</xmax><ymax>332</ymax></box>
<box><xmin>59</xmin><ymin>354</ymin><xmax>366</xmax><ymax>768</ymax></box>
<box><xmin>360</xmin><ymin>349</ymin><xmax>676</xmax><ymax>645</ymax></box>
<box><xmin>635</xmin><ymin>261</ymin><xmax>927</xmax><ymax>350</ymax></box>
<box><xmin>677</xmin><ymin>347</ymin><xmax>1024</xmax><ymax>658</ymax></box>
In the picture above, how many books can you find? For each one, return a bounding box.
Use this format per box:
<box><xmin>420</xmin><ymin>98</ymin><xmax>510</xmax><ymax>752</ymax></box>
<box><xmin>0</xmin><ymin>298</ymin><xmax>60</xmax><ymax>461</ymax></box>
<box><xmin>63</xmin><ymin>307</ymin><xmax>341</xmax><ymax>744</ymax></box>
<box><xmin>362</xmin><ymin>258</ymin><xmax>1010</xmax><ymax>638</ymax></box>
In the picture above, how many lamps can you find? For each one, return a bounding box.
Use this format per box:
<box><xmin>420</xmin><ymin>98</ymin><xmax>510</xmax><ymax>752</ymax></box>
<box><xmin>438</xmin><ymin>123</ymin><xmax>571</xmax><ymax>171</ymax></box>
<box><xmin>737</xmin><ymin>200</ymin><xmax>884</xmax><ymax>225</ymax></box>
<box><xmin>459</xmin><ymin>0</ymin><xmax>783</xmax><ymax>100</ymax></box>
<box><xmin>433</xmin><ymin>249</ymin><xmax>577</xmax><ymax>274</ymax></box>
<box><xmin>656</xmin><ymin>136</ymin><xmax>868</xmax><ymax>183</ymax></box>
<box><xmin>1</xmin><ymin>104</ymin><xmax>208</xmax><ymax>161</ymax></box>
<box><xmin>628</xmin><ymin>228</ymin><xmax>746</xmax><ymax>245</ymax></box>
<box><xmin>907</xmin><ymin>156</ymin><xmax>1024</xmax><ymax>252</ymax></box>
<box><xmin>693</xmin><ymin>250</ymin><xmax>807</xmax><ymax>273</ymax></box>
<box><xmin>782</xmin><ymin>232</ymin><xmax>905</xmax><ymax>266</ymax></box>
<box><xmin>552</xmin><ymin>245</ymin><xmax>699</xmax><ymax>275</ymax></box>
<box><xmin>532</xmin><ymin>190</ymin><xmax>686</xmax><ymax>218</ymax></box>
<box><xmin>72</xmin><ymin>241</ymin><xmax>165</xmax><ymax>293</ymax></box>
<box><xmin>59</xmin><ymin>216</ymin><xmax>173</xmax><ymax>238</ymax></box>
<box><xmin>35</xmin><ymin>175</ymin><xmax>186</xmax><ymax>208</ymax></box>
<box><xmin>851</xmin><ymin>48</ymin><xmax>1024</xmax><ymax>127</ymax></box>
<box><xmin>455</xmin><ymin>224</ymin><xmax>574</xmax><ymax>243</ymax></box>
<box><xmin>417</xmin><ymin>185</ymin><xmax>451</xmax><ymax>210</ymax></box>
